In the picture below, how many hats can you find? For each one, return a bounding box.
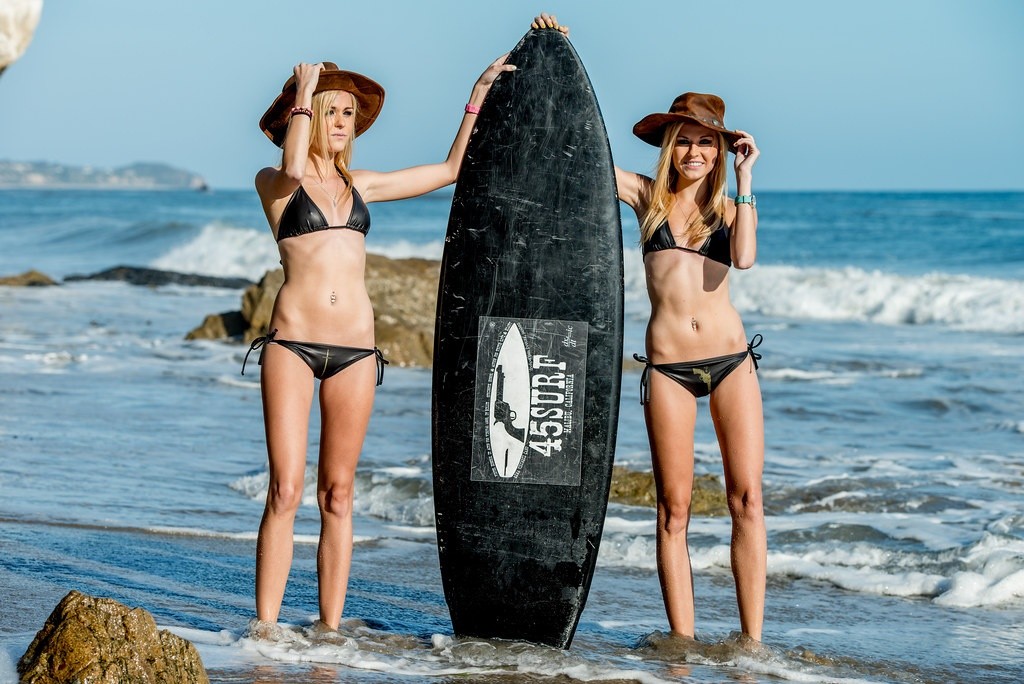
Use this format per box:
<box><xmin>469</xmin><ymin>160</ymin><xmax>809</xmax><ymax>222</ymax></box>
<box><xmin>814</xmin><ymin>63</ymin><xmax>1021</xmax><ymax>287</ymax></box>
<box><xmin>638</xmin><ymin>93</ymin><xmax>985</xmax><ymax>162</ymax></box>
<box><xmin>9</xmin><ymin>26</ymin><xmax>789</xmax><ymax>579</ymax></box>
<box><xmin>259</xmin><ymin>61</ymin><xmax>386</xmax><ymax>150</ymax></box>
<box><xmin>632</xmin><ymin>92</ymin><xmax>747</xmax><ymax>155</ymax></box>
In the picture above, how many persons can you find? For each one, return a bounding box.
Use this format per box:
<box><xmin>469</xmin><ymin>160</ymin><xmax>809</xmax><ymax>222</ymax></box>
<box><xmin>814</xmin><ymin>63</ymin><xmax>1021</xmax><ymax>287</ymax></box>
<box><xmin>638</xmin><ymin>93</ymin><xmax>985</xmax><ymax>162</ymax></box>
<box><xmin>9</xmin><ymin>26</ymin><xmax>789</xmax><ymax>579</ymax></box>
<box><xmin>255</xmin><ymin>52</ymin><xmax>519</xmax><ymax>632</ymax></box>
<box><xmin>531</xmin><ymin>12</ymin><xmax>768</xmax><ymax>644</ymax></box>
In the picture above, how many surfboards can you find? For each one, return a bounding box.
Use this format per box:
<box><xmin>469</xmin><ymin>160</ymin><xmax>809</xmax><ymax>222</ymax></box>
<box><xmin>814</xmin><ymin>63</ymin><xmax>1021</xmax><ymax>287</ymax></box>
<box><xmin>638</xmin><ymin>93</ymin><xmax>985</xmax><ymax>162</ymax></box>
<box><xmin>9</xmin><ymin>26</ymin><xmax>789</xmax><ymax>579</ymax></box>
<box><xmin>429</xmin><ymin>26</ymin><xmax>625</xmax><ymax>646</ymax></box>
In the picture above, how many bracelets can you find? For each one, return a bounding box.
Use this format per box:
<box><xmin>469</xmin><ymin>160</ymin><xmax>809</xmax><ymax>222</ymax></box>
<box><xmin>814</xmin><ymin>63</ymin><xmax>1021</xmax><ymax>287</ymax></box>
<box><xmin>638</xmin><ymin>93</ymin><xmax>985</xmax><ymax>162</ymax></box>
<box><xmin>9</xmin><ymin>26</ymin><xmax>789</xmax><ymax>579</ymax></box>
<box><xmin>465</xmin><ymin>103</ymin><xmax>481</xmax><ymax>115</ymax></box>
<box><xmin>291</xmin><ymin>106</ymin><xmax>313</xmax><ymax>121</ymax></box>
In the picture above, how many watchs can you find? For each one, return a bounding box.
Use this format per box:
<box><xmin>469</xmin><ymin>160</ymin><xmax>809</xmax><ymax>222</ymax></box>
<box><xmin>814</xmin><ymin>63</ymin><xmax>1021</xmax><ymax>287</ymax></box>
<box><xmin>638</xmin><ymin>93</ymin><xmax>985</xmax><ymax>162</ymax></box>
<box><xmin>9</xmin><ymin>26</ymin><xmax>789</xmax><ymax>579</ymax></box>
<box><xmin>734</xmin><ymin>195</ymin><xmax>756</xmax><ymax>209</ymax></box>
